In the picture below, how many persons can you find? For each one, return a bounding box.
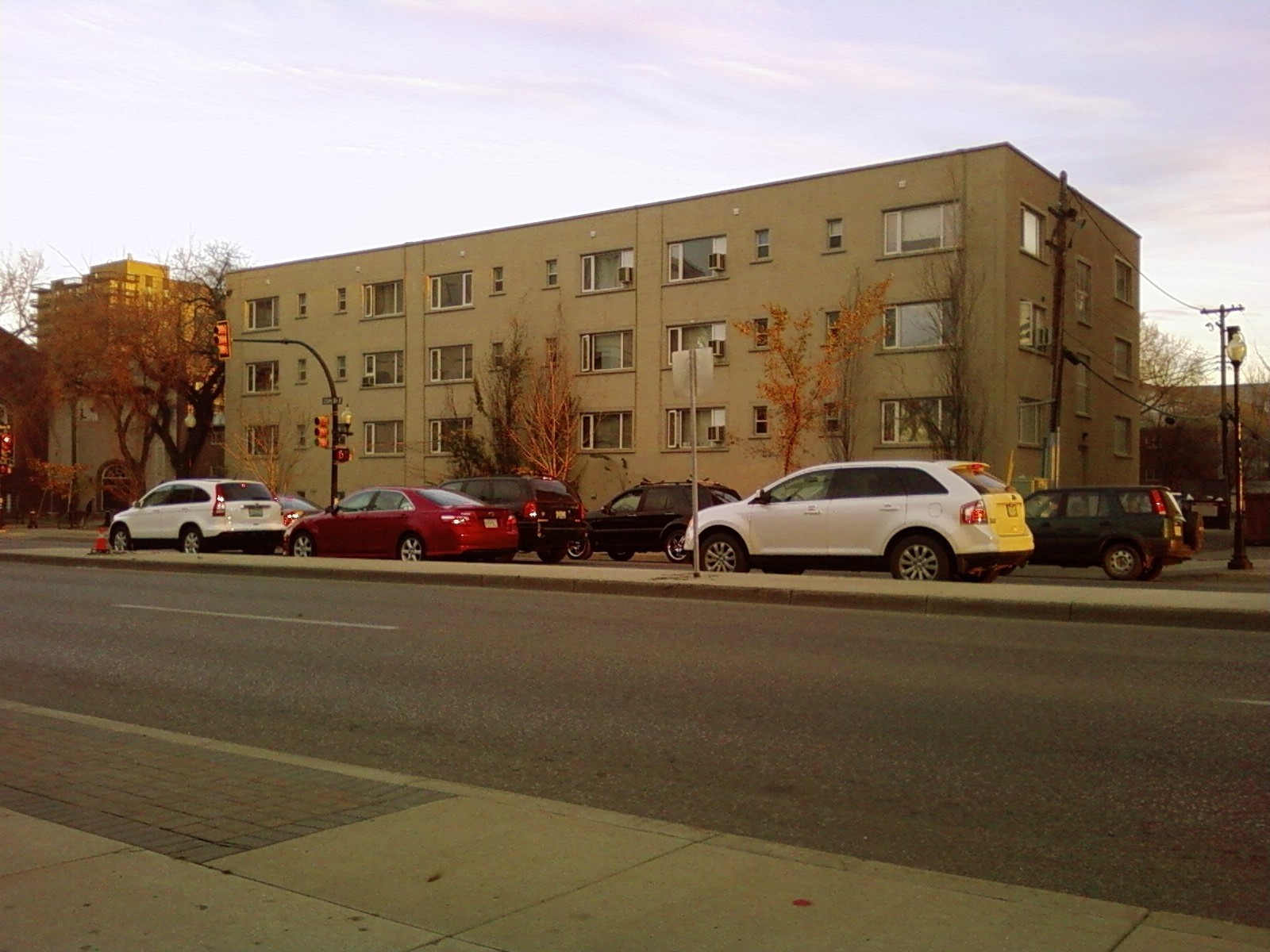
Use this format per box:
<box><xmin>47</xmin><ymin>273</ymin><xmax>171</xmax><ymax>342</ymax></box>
<box><xmin>793</xmin><ymin>475</ymin><xmax>833</xmax><ymax>500</ymax></box>
<box><xmin>84</xmin><ymin>497</ymin><xmax>96</xmax><ymax>520</ymax></box>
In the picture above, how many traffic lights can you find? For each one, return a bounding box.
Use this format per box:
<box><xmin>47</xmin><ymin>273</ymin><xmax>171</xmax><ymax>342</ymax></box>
<box><xmin>314</xmin><ymin>415</ymin><xmax>330</xmax><ymax>449</ymax></box>
<box><xmin>335</xmin><ymin>448</ymin><xmax>350</xmax><ymax>462</ymax></box>
<box><xmin>214</xmin><ymin>321</ymin><xmax>232</xmax><ymax>360</ymax></box>
<box><xmin>0</xmin><ymin>432</ymin><xmax>16</xmax><ymax>468</ymax></box>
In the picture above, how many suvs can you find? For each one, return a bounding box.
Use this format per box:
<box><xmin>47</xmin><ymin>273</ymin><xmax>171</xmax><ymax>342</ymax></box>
<box><xmin>682</xmin><ymin>456</ymin><xmax>1035</xmax><ymax>583</ymax></box>
<box><xmin>567</xmin><ymin>476</ymin><xmax>743</xmax><ymax>564</ymax></box>
<box><xmin>436</xmin><ymin>473</ymin><xmax>593</xmax><ymax>563</ymax></box>
<box><xmin>106</xmin><ymin>478</ymin><xmax>288</xmax><ymax>555</ymax></box>
<box><xmin>997</xmin><ymin>484</ymin><xmax>1206</xmax><ymax>582</ymax></box>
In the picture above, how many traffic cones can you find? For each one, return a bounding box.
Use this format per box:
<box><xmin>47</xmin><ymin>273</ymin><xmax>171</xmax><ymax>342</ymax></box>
<box><xmin>95</xmin><ymin>527</ymin><xmax>108</xmax><ymax>556</ymax></box>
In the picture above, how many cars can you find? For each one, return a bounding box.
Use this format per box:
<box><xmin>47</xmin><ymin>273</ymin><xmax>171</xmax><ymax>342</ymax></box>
<box><xmin>274</xmin><ymin>493</ymin><xmax>324</xmax><ymax>526</ymax></box>
<box><xmin>284</xmin><ymin>486</ymin><xmax>518</xmax><ymax>562</ymax></box>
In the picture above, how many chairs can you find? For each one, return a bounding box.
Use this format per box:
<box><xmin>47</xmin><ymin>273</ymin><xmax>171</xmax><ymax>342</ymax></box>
<box><xmin>842</xmin><ymin>476</ymin><xmax>864</xmax><ymax>498</ymax></box>
<box><xmin>374</xmin><ymin>494</ymin><xmax>397</xmax><ymax>510</ymax></box>
<box><xmin>1071</xmin><ymin>497</ymin><xmax>1089</xmax><ymax>516</ymax></box>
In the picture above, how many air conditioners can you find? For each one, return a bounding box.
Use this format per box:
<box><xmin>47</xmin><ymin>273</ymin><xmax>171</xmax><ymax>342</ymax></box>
<box><xmin>707</xmin><ymin>338</ymin><xmax>724</xmax><ymax>358</ymax></box>
<box><xmin>706</xmin><ymin>250</ymin><xmax>725</xmax><ymax>272</ymax></box>
<box><xmin>362</xmin><ymin>375</ymin><xmax>374</xmax><ymax>387</ymax></box>
<box><xmin>617</xmin><ymin>265</ymin><xmax>632</xmax><ymax>284</ymax></box>
<box><xmin>706</xmin><ymin>424</ymin><xmax>724</xmax><ymax>444</ymax></box>
<box><xmin>1035</xmin><ymin>325</ymin><xmax>1053</xmax><ymax>348</ymax></box>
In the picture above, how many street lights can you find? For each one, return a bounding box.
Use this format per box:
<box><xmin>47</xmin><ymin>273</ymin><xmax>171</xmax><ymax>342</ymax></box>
<box><xmin>1226</xmin><ymin>332</ymin><xmax>1253</xmax><ymax>570</ymax></box>
